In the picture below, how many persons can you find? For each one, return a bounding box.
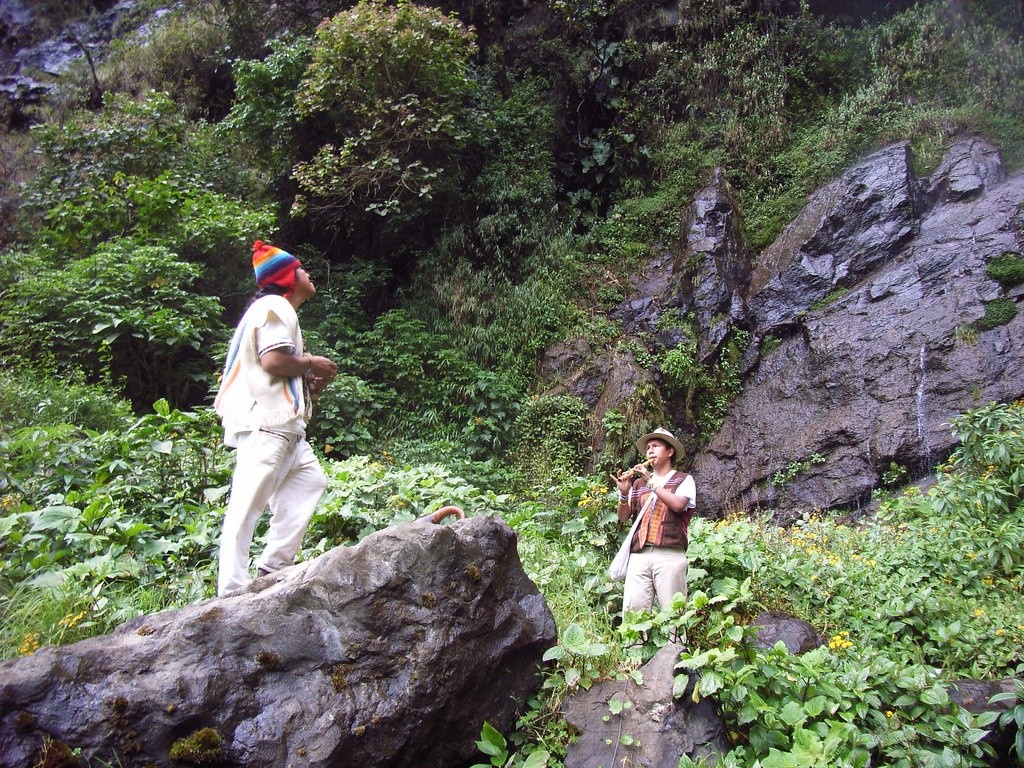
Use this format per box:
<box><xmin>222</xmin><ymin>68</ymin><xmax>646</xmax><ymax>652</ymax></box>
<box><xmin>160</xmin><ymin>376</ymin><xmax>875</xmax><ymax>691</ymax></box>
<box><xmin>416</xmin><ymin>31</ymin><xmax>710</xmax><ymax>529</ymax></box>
<box><xmin>607</xmin><ymin>425</ymin><xmax>695</xmax><ymax>670</ymax></box>
<box><xmin>214</xmin><ymin>240</ymin><xmax>337</xmax><ymax>597</ymax></box>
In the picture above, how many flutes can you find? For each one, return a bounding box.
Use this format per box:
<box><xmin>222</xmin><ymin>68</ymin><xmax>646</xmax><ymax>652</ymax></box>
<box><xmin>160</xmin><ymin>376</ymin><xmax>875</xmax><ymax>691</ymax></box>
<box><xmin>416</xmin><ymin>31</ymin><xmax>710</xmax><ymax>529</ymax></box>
<box><xmin>618</xmin><ymin>458</ymin><xmax>655</xmax><ymax>483</ymax></box>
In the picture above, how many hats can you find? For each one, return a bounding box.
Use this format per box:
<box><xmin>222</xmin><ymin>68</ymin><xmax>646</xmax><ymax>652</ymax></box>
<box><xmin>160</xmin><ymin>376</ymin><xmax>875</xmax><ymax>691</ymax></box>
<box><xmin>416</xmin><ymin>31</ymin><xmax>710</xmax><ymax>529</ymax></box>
<box><xmin>253</xmin><ymin>239</ymin><xmax>301</xmax><ymax>298</ymax></box>
<box><xmin>636</xmin><ymin>427</ymin><xmax>684</xmax><ymax>462</ymax></box>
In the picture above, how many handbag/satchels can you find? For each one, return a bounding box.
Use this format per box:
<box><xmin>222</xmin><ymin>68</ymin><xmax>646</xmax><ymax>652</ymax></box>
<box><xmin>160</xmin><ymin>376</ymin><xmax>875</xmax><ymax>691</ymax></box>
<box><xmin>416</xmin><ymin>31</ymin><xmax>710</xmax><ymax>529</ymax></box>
<box><xmin>609</xmin><ymin>539</ymin><xmax>630</xmax><ymax>581</ymax></box>
